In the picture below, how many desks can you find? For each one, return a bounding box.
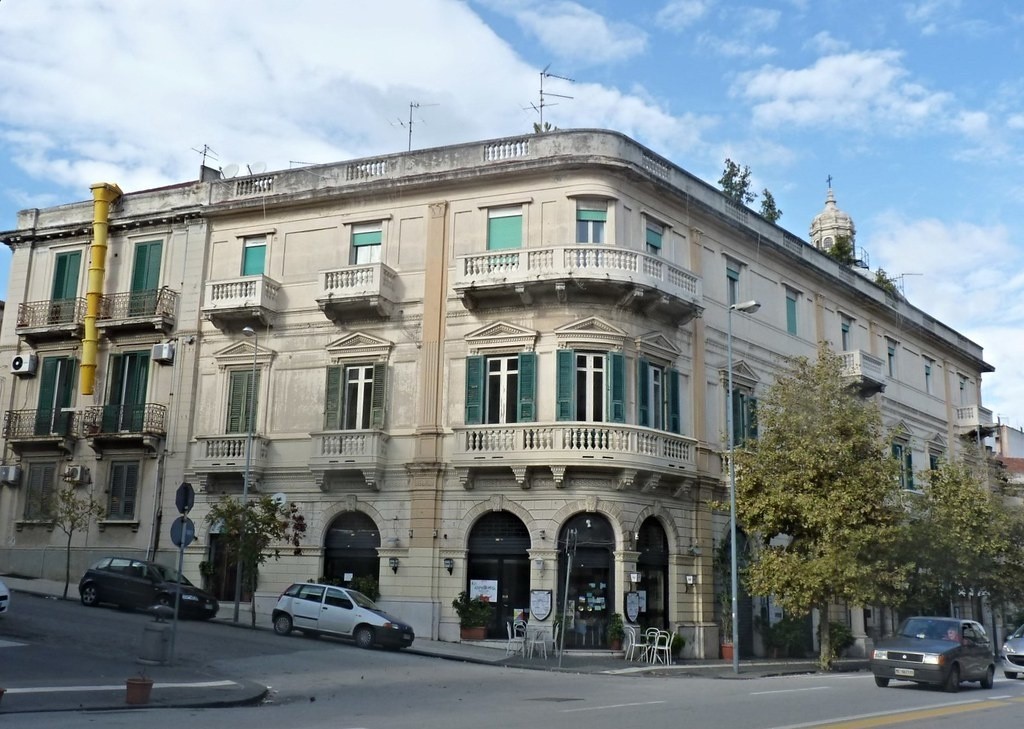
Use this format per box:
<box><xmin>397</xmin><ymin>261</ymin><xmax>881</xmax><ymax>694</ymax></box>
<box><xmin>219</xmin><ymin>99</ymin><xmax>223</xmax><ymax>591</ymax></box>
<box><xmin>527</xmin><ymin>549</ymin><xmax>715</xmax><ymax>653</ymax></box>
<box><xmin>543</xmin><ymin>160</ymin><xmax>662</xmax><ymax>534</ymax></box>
<box><xmin>639</xmin><ymin>634</ymin><xmax>666</xmax><ymax>661</ymax></box>
<box><xmin>521</xmin><ymin>629</ymin><xmax>552</xmax><ymax>658</ymax></box>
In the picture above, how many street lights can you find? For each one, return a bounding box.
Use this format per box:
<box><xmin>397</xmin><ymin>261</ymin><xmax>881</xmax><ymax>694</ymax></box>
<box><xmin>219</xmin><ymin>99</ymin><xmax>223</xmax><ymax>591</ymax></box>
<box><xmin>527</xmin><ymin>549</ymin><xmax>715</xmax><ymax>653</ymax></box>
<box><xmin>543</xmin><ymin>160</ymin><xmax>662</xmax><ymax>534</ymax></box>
<box><xmin>727</xmin><ymin>300</ymin><xmax>761</xmax><ymax>673</ymax></box>
<box><xmin>233</xmin><ymin>326</ymin><xmax>258</xmax><ymax>624</ymax></box>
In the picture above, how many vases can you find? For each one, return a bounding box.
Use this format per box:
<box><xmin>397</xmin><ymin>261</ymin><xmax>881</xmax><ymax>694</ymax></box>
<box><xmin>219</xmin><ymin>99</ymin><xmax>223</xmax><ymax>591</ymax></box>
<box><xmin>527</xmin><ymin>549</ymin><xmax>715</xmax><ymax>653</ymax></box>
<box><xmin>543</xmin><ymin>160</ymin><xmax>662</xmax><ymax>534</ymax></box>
<box><xmin>125</xmin><ymin>677</ymin><xmax>155</xmax><ymax>703</ymax></box>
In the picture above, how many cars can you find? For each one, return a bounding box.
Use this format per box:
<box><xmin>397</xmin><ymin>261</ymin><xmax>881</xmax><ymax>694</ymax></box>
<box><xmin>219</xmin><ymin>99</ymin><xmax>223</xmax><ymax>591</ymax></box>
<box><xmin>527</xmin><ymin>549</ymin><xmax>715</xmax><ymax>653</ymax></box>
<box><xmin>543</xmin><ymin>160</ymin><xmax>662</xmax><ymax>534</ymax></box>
<box><xmin>78</xmin><ymin>556</ymin><xmax>220</xmax><ymax>621</ymax></box>
<box><xmin>870</xmin><ymin>616</ymin><xmax>996</xmax><ymax>692</ymax></box>
<box><xmin>271</xmin><ymin>582</ymin><xmax>415</xmax><ymax>649</ymax></box>
<box><xmin>1001</xmin><ymin>624</ymin><xmax>1024</xmax><ymax>679</ymax></box>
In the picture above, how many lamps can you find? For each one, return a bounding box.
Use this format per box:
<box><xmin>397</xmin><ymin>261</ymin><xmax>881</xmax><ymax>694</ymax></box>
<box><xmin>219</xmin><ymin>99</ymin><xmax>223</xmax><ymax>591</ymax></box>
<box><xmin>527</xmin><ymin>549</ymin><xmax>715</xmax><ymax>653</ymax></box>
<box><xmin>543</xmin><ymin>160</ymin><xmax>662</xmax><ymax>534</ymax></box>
<box><xmin>444</xmin><ymin>558</ymin><xmax>453</xmax><ymax>576</ymax></box>
<box><xmin>388</xmin><ymin>557</ymin><xmax>398</xmax><ymax>574</ymax></box>
<box><xmin>493</xmin><ymin>534</ymin><xmax>503</xmax><ymax>543</ymax></box>
<box><xmin>536</xmin><ymin>557</ymin><xmax>545</xmax><ymax>576</ymax></box>
<box><xmin>628</xmin><ymin>571</ymin><xmax>641</xmax><ymax>584</ymax></box>
<box><xmin>684</xmin><ymin>573</ymin><xmax>696</xmax><ymax>593</ymax></box>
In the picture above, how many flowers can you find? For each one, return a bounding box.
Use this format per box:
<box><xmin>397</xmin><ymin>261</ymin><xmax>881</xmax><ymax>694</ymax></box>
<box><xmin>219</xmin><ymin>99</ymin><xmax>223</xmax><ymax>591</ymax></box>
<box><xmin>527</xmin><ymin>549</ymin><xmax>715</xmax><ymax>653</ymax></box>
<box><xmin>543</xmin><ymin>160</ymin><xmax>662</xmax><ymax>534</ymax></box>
<box><xmin>479</xmin><ymin>593</ymin><xmax>490</xmax><ymax>602</ymax></box>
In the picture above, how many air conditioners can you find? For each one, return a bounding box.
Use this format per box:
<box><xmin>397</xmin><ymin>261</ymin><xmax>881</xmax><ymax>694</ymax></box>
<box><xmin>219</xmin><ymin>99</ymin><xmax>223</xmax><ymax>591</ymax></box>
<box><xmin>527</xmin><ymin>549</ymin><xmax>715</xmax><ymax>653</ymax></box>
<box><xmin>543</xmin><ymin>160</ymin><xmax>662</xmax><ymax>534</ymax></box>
<box><xmin>0</xmin><ymin>466</ymin><xmax>21</xmax><ymax>486</ymax></box>
<box><xmin>152</xmin><ymin>343</ymin><xmax>173</xmax><ymax>363</ymax></box>
<box><xmin>10</xmin><ymin>354</ymin><xmax>38</xmax><ymax>377</ymax></box>
<box><xmin>64</xmin><ymin>466</ymin><xmax>87</xmax><ymax>483</ymax></box>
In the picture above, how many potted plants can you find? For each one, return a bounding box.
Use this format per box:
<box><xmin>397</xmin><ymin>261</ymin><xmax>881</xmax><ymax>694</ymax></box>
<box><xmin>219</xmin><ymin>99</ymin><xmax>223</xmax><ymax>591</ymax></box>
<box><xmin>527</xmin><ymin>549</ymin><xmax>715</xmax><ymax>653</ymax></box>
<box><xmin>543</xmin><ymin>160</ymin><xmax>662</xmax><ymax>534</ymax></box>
<box><xmin>719</xmin><ymin>590</ymin><xmax>738</xmax><ymax>660</ymax></box>
<box><xmin>87</xmin><ymin>408</ymin><xmax>101</xmax><ymax>433</ymax></box>
<box><xmin>452</xmin><ymin>592</ymin><xmax>494</xmax><ymax>638</ymax></box>
<box><xmin>607</xmin><ymin>613</ymin><xmax>625</xmax><ymax>650</ymax></box>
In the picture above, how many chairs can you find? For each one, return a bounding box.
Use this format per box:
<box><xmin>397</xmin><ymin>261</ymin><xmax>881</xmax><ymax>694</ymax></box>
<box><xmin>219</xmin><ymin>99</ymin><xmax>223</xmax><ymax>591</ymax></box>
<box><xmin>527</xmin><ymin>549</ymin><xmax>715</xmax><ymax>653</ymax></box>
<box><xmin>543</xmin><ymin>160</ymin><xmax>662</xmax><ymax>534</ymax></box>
<box><xmin>651</xmin><ymin>631</ymin><xmax>670</xmax><ymax>666</ymax></box>
<box><xmin>624</xmin><ymin>626</ymin><xmax>649</xmax><ymax>664</ymax></box>
<box><xmin>651</xmin><ymin>631</ymin><xmax>676</xmax><ymax>663</ymax></box>
<box><xmin>529</xmin><ymin>629</ymin><xmax>548</xmax><ymax>660</ymax></box>
<box><xmin>506</xmin><ymin>622</ymin><xmax>525</xmax><ymax>657</ymax></box>
<box><xmin>544</xmin><ymin>623</ymin><xmax>561</xmax><ymax>658</ymax></box>
<box><xmin>642</xmin><ymin>625</ymin><xmax>661</xmax><ymax>663</ymax></box>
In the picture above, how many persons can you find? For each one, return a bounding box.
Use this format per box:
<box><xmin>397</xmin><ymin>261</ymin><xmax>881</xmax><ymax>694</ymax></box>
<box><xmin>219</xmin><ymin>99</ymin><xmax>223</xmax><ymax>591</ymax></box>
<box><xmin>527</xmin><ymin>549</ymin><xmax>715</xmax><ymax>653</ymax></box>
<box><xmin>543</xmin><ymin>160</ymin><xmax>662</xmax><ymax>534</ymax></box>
<box><xmin>942</xmin><ymin>626</ymin><xmax>960</xmax><ymax>642</ymax></box>
